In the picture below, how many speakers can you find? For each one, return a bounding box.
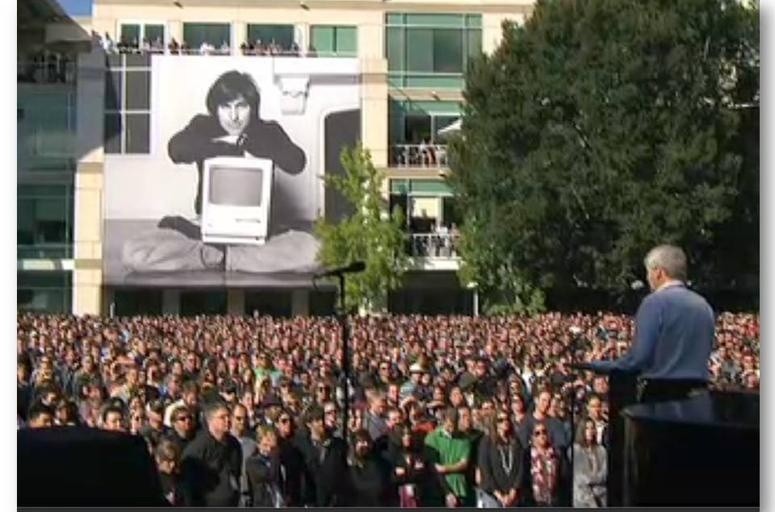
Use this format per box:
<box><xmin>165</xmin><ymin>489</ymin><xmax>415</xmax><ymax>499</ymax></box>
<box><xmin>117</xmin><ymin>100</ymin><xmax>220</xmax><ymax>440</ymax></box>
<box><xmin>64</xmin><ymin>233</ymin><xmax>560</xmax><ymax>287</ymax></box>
<box><xmin>18</xmin><ymin>426</ymin><xmax>174</xmax><ymax>506</ymax></box>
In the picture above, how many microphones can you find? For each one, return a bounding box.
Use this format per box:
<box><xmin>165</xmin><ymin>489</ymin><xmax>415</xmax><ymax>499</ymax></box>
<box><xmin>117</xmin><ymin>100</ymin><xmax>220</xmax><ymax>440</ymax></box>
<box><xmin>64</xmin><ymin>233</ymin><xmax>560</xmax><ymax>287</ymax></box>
<box><xmin>325</xmin><ymin>261</ymin><xmax>365</xmax><ymax>276</ymax></box>
<box><xmin>626</xmin><ymin>280</ymin><xmax>645</xmax><ymax>296</ymax></box>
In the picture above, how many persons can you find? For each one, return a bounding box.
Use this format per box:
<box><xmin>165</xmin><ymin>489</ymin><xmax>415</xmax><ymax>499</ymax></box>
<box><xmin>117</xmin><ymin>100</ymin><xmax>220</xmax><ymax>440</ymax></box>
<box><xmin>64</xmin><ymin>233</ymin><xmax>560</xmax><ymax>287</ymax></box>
<box><xmin>562</xmin><ymin>241</ymin><xmax>715</xmax><ymax>404</ymax></box>
<box><xmin>415</xmin><ymin>236</ymin><xmax>459</xmax><ymax>256</ymax></box>
<box><xmin>121</xmin><ymin>69</ymin><xmax>326</xmax><ymax>275</ymax></box>
<box><xmin>90</xmin><ymin>29</ymin><xmax>319</xmax><ymax>58</ymax></box>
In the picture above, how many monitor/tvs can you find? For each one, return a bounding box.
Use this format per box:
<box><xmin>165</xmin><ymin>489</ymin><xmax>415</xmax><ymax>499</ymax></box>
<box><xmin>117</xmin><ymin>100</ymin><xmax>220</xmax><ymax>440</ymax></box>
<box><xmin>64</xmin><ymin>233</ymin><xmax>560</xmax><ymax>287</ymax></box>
<box><xmin>201</xmin><ymin>156</ymin><xmax>274</xmax><ymax>244</ymax></box>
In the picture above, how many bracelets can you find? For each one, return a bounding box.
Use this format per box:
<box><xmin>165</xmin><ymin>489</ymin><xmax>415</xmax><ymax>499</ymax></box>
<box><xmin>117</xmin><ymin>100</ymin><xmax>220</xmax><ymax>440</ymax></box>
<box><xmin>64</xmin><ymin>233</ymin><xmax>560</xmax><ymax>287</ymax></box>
<box><xmin>236</xmin><ymin>132</ymin><xmax>250</xmax><ymax>148</ymax></box>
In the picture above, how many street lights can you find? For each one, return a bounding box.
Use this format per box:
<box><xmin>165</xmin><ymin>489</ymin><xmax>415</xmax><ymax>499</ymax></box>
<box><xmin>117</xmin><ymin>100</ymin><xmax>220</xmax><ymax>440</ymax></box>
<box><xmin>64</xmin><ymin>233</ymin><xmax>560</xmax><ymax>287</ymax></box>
<box><xmin>466</xmin><ymin>281</ymin><xmax>480</xmax><ymax>316</ymax></box>
